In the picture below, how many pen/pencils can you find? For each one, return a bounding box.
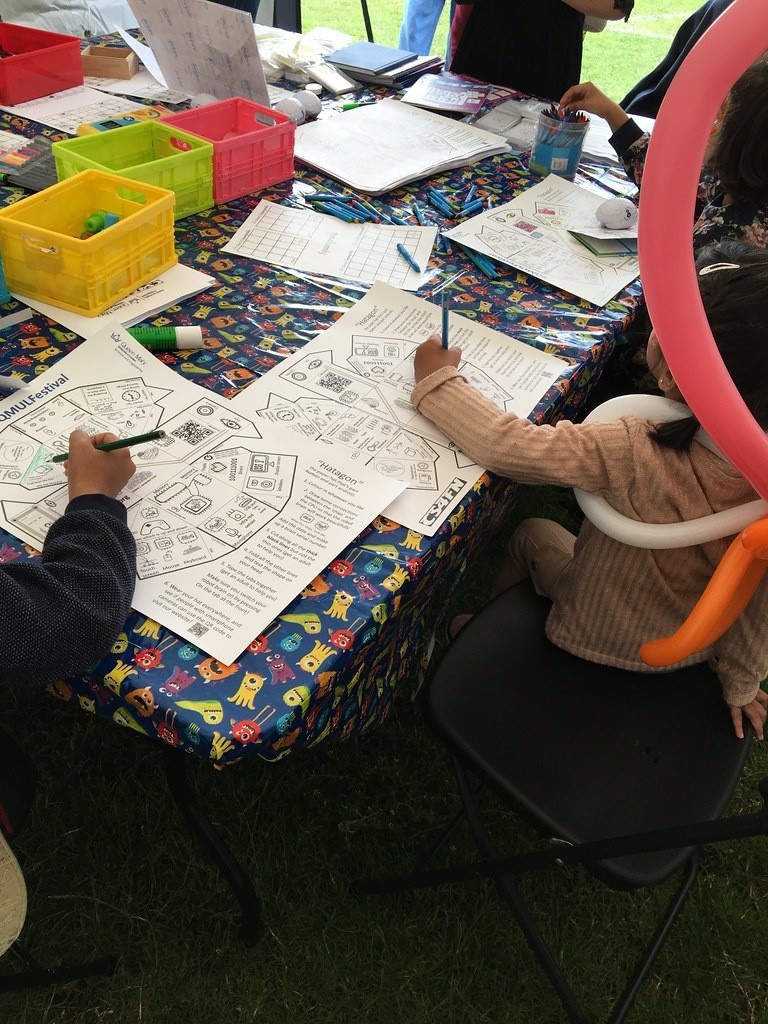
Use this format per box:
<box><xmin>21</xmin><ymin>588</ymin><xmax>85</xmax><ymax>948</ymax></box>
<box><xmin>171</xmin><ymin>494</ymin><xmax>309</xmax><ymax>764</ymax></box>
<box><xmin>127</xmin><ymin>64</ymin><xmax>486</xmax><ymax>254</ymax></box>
<box><xmin>46</xmin><ymin>430</ymin><xmax>167</xmax><ymax>463</ymax></box>
<box><xmin>456</xmin><ymin>243</ymin><xmax>493</xmax><ymax>280</ymax></box>
<box><xmin>442</xmin><ymin>292</ymin><xmax>449</xmax><ymax>351</ymax></box>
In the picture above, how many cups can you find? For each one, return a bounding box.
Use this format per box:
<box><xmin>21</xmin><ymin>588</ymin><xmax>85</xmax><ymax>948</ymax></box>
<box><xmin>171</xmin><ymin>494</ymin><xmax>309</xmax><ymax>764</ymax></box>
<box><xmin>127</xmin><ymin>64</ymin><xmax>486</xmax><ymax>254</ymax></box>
<box><xmin>529</xmin><ymin>107</ymin><xmax>591</xmax><ymax>181</ymax></box>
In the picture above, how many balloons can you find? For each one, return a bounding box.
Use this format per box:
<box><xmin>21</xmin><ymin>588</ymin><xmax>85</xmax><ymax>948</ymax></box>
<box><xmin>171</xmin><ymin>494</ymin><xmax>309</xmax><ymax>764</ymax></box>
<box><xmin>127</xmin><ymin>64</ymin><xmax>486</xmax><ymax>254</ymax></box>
<box><xmin>576</xmin><ymin>0</ymin><xmax>768</xmax><ymax>548</ymax></box>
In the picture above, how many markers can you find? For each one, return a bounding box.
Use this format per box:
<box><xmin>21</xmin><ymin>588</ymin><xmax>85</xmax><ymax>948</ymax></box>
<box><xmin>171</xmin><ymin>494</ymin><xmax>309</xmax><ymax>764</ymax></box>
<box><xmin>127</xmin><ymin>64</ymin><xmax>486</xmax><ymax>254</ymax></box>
<box><xmin>342</xmin><ymin>102</ymin><xmax>377</xmax><ymax>110</ymax></box>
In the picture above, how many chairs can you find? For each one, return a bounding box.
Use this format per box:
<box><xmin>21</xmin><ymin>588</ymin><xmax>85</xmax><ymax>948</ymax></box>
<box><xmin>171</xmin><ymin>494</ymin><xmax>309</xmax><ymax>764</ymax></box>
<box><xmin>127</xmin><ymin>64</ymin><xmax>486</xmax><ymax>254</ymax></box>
<box><xmin>348</xmin><ymin>578</ymin><xmax>767</xmax><ymax>1020</ymax></box>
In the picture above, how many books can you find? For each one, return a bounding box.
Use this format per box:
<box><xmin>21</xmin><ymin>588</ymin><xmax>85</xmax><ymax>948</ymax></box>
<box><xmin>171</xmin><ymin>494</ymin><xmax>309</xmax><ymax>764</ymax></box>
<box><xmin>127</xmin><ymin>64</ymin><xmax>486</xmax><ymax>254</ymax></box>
<box><xmin>323</xmin><ymin>40</ymin><xmax>446</xmax><ymax>91</ymax></box>
<box><xmin>400</xmin><ymin>70</ymin><xmax>521</xmax><ymax>114</ymax></box>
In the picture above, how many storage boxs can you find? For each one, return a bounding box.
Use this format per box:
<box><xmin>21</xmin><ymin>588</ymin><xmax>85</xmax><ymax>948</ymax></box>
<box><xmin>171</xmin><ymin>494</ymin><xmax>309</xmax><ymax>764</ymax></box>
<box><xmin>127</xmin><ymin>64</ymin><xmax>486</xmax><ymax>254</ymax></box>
<box><xmin>157</xmin><ymin>96</ymin><xmax>298</xmax><ymax>206</ymax></box>
<box><xmin>51</xmin><ymin>119</ymin><xmax>215</xmax><ymax>223</ymax></box>
<box><xmin>0</xmin><ymin>21</ymin><xmax>84</xmax><ymax>106</ymax></box>
<box><xmin>0</xmin><ymin>170</ymin><xmax>179</xmax><ymax>316</ymax></box>
<box><xmin>80</xmin><ymin>45</ymin><xmax>141</xmax><ymax>80</ymax></box>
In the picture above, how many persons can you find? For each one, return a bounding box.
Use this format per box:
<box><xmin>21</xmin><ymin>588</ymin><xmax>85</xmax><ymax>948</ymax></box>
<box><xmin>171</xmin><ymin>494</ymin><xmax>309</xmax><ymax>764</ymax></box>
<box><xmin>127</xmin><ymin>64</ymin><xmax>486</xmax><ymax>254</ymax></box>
<box><xmin>619</xmin><ymin>0</ymin><xmax>733</xmax><ymax>121</ymax></box>
<box><xmin>397</xmin><ymin>0</ymin><xmax>457</xmax><ymax>72</ymax></box>
<box><xmin>0</xmin><ymin>430</ymin><xmax>137</xmax><ymax>841</ymax></box>
<box><xmin>448</xmin><ymin>0</ymin><xmax>634</xmax><ymax>103</ymax></box>
<box><xmin>560</xmin><ymin>61</ymin><xmax>768</xmax><ymax>398</ymax></box>
<box><xmin>410</xmin><ymin>236</ymin><xmax>768</xmax><ymax>740</ymax></box>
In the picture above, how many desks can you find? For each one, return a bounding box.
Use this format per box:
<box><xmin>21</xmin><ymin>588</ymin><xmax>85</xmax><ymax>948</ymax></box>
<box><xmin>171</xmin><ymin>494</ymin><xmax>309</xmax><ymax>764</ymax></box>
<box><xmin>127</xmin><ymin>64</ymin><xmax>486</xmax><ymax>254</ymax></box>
<box><xmin>1</xmin><ymin>26</ymin><xmax>655</xmax><ymax>942</ymax></box>
